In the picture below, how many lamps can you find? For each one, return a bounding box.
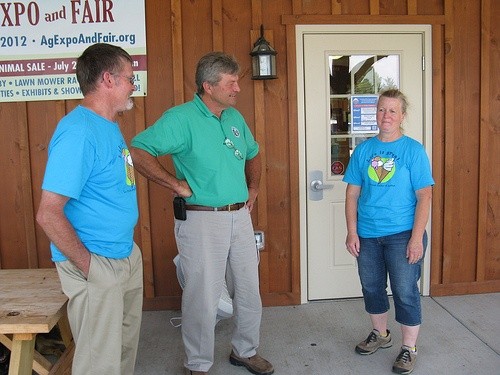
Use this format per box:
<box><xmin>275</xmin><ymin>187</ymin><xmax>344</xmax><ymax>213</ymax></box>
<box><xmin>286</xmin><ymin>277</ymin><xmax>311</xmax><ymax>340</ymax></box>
<box><xmin>248</xmin><ymin>22</ymin><xmax>279</xmax><ymax>81</ymax></box>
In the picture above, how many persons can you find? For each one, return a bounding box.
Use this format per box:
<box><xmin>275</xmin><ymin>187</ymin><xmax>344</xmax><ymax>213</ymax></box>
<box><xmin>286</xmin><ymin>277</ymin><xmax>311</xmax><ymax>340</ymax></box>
<box><xmin>130</xmin><ymin>51</ymin><xmax>275</xmax><ymax>375</ymax></box>
<box><xmin>342</xmin><ymin>89</ymin><xmax>435</xmax><ymax>375</ymax></box>
<box><xmin>36</xmin><ymin>43</ymin><xmax>143</xmax><ymax>375</ymax></box>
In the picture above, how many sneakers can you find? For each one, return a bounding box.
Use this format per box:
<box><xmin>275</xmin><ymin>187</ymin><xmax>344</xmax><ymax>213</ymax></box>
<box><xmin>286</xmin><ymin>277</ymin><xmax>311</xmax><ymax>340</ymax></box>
<box><xmin>355</xmin><ymin>328</ymin><xmax>392</xmax><ymax>355</ymax></box>
<box><xmin>392</xmin><ymin>345</ymin><xmax>418</xmax><ymax>375</ymax></box>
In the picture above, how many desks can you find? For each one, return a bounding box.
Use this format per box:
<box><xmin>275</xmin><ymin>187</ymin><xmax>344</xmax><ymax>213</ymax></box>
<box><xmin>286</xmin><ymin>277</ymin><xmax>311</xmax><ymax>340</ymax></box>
<box><xmin>0</xmin><ymin>267</ymin><xmax>76</xmax><ymax>375</ymax></box>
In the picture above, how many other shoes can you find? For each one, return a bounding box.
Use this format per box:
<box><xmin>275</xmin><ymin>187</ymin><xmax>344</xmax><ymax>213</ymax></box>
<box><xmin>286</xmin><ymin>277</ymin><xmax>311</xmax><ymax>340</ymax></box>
<box><xmin>184</xmin><ymin>366</ymin><xmax>207</xmax><ymax>375</ymax></box>
<box><xmin>229</xmin><ymin>351</ymin><xmax>274</xmax><ymax>375</ymax></box>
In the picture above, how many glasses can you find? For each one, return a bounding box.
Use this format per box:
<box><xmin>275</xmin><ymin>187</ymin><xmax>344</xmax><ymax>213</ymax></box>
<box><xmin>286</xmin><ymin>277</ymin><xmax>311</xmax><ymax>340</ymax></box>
<box><xmin>110</xmin><ymin>72</ymin><xmax>136</xmax><ymax>85</ymax></box>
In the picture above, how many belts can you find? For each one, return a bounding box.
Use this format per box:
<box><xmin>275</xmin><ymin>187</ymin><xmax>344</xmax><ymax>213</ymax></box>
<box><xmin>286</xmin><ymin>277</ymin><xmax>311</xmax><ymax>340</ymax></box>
<box><xmin>185</xmin><ymin>201</ymin><xmax>250</xmax><ymax>211</ymax></box>
